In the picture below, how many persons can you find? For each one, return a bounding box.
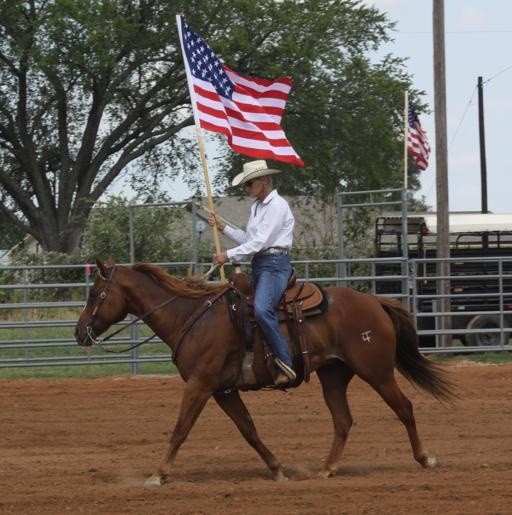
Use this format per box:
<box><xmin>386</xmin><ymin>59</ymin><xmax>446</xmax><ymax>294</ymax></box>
<box><xmin>208</xmin><ymin>159</ymin><xmax>297</xmax><ymax>388</ymax></box>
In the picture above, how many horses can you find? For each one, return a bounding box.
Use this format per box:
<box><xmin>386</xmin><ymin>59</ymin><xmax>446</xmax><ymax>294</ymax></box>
<box><xmin>72</xmin><ymin>254</ymin><xmax>466</xmax><ymax>492</ymax></box>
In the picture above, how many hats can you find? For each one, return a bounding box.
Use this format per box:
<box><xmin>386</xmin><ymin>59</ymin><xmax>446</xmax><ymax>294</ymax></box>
<box><xmin>231</xmin><ymin>158</ymin><xmax>281</xmax><ymax>186</ymax></box>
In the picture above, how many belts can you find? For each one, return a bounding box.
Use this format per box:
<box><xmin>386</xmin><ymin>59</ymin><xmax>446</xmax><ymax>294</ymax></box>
<box><xmin>259</xmin><ymin>248</ymin><xmax>289</xmax><ymax>256</ymax></box>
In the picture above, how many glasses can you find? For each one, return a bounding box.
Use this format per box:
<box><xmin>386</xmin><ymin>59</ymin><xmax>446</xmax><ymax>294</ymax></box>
<box><xmin>245</xmin><ymin>182</ymin><xmax>255</xmax><ymax>187</ymax></box>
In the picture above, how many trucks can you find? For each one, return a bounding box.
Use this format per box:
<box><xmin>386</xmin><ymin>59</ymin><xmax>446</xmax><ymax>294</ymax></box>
<box><xmin>373</xmin><ymin>218</ymin><xmax>512</xmax><ymax>354</ymax></box>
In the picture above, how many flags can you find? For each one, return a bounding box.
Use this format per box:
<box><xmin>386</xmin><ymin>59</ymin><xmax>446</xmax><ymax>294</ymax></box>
<box><xmin>406</xmin><ymin>96</ymin><xmax>431</xmax><ymax>173</ymax></box>
<box><xmin>174</xmin><ymin>14</ymin><xmax>305</xmax><ymax>166</ymax></box>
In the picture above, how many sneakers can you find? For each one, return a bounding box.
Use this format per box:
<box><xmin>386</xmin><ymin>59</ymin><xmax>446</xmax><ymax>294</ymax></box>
<box><xmin>274</xmin><ymin>371</ymin><xmax>291</xmax><ymax>385</ymax></box>
